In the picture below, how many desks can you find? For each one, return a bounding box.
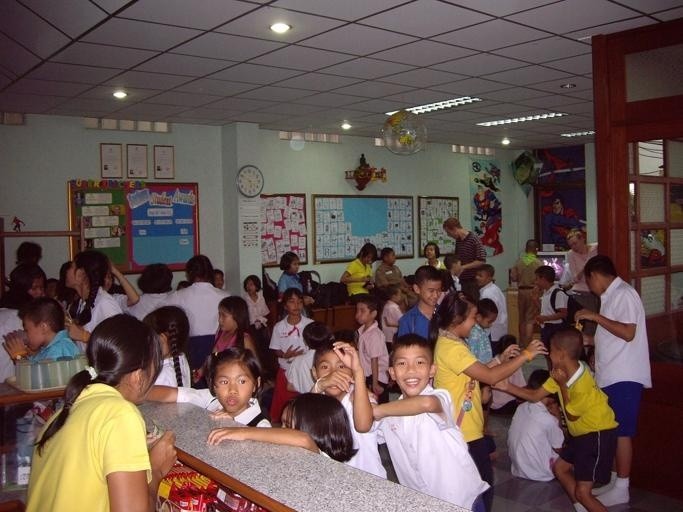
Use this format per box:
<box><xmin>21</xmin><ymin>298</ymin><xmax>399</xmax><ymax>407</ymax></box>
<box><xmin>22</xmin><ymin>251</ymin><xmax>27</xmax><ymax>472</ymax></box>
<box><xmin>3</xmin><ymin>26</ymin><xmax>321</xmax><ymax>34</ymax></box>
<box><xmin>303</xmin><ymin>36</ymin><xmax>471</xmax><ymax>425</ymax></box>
<box><xmin>0</xmin><ymin>378</ymin><xmax>66</xmax><ymax>408</ymax></box>
<box><xmin>135</xmin><ymin>399</ymin><xmax>474</xmax><ymax>510</ymax></box>
<box><xmin>314</xmin><ymin>305</ymin><xmax>359</xmax><ymax>335</ymax></box>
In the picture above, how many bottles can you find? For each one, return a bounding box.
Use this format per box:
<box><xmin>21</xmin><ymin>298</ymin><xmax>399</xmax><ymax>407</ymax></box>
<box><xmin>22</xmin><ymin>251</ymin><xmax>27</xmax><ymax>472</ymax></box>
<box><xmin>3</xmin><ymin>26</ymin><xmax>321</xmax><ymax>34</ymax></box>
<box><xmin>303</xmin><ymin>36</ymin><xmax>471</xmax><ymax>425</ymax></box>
<box><xmin>305</xmin><ymin>280</ymin><xmax>311</xmax><ymax>294</ymax></box>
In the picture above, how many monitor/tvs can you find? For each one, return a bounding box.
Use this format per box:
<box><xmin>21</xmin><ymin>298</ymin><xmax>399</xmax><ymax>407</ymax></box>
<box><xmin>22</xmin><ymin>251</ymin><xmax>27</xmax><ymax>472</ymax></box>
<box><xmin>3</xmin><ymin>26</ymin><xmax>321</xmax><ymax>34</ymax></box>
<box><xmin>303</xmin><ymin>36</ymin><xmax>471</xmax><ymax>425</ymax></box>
<box><xmin>536</xmin><ymin>252</ymin><xmax>567</xmax><ymax>281</ymax></box>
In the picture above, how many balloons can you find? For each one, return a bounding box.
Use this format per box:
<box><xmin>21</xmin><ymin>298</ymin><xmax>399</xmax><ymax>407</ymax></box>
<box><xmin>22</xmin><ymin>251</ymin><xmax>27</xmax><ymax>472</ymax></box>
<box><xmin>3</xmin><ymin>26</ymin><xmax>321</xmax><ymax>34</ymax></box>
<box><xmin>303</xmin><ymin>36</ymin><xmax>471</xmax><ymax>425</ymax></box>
<box><xmin>382</xmin><ymin>109</ymin><xmax>427</xmax><ymax>155</ymax></box>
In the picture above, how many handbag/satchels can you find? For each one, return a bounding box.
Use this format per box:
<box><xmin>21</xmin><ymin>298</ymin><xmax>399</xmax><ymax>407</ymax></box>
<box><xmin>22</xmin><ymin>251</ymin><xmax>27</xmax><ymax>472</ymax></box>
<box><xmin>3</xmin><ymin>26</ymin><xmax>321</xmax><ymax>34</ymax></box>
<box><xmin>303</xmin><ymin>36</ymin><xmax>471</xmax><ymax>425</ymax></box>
<box><xmin>311</xmin><ymin>281</ymin><xmax>348</xmax><ymax>308</ymax></box>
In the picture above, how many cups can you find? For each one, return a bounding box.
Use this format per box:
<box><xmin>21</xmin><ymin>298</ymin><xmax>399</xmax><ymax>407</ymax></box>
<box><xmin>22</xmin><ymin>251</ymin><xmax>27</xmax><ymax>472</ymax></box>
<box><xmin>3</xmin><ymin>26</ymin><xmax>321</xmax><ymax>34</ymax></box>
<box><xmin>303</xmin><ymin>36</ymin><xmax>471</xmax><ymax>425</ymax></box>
<box><xmin>14</xmin><ymin>353</ymin><xmax>89</xmax><ymax>390</ymax></box>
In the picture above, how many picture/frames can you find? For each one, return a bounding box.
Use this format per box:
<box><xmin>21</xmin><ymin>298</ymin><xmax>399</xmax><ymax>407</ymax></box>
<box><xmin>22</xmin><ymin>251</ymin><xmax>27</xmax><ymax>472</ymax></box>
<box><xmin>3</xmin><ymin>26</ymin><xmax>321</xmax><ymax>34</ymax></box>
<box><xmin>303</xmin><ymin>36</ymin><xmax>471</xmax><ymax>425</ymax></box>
<box><xmin>125</xmin><ymin>143</ymin><xmax>149</xmax><ymax>180</ymax></box>
<box><xmin>153</xmin><ymin>144</ymin><xmax>175</xmax><ymax>180</ymax></box>
<box><xmin>98</xmin><ymin>140</ymin><xmax>124</xmax><ymax>180</ymax></box>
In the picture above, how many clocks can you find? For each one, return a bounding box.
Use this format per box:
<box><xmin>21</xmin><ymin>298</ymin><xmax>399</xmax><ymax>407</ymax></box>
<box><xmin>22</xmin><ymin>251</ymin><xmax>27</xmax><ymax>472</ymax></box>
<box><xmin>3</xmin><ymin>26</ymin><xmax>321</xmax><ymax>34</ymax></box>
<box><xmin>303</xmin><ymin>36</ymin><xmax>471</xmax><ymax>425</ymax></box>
<box><xmin>236</xmin><ymin>164</ymin><xmax>266</xmax><ymax>198</ymax></box>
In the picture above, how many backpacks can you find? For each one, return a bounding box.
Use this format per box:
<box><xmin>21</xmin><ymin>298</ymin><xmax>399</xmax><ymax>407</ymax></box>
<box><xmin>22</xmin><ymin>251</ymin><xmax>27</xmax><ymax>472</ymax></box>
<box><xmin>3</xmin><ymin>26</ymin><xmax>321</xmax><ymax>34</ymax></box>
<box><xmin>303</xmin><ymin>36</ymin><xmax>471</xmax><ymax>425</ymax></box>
<box><xmin>550</xmin><ymin>288</ymin><xmax>584</xmax><ymax>324</ymax></box>
